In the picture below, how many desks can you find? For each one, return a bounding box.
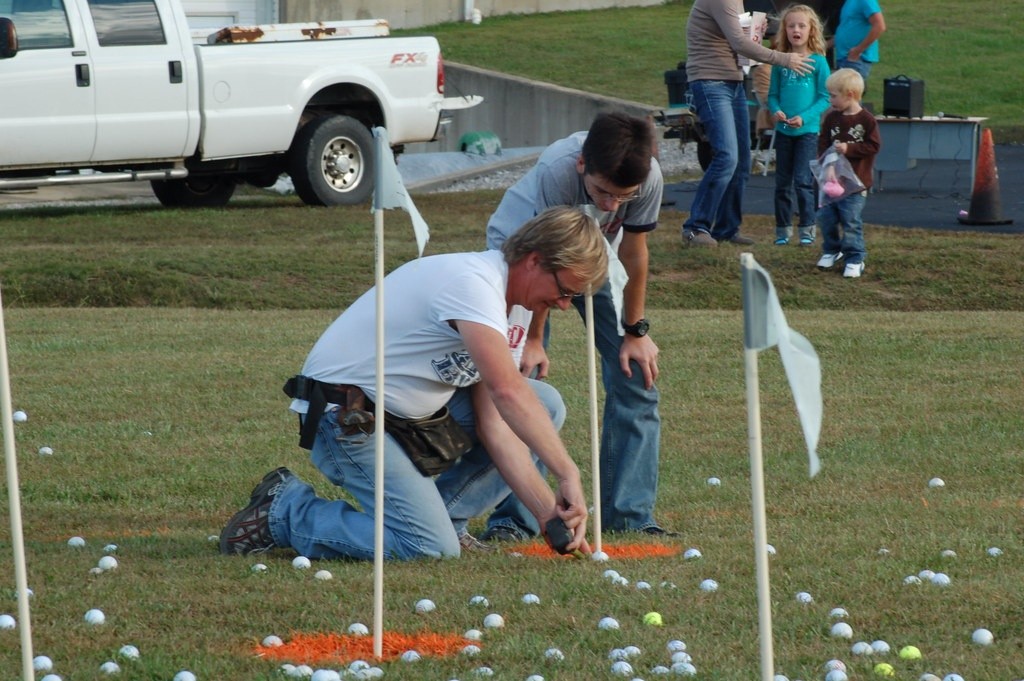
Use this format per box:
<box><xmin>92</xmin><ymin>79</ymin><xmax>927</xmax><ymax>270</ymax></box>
<box><xmin>869</xmin><ymin>116</ymin><xmax>990</xmax><ymax>201</ymax></box>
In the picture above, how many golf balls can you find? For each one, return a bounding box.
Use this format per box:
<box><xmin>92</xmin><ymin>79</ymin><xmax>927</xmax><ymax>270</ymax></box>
<box><xmin>84</xmin><ymin>609</ymin><xmax>106</xmax><ymax>625</ymax></box>
<box><xmin>39</xmin><ymin>446</ymin><xmax>53</xmax><ymax>455</ymax></box>
<box><xmin>33</xmin><ymin>655</ymin><xmax>53</xmax><ymax>671</ymax></box>
<box><xmin>89</xmin><ymin>556</ymin><xmax>118</xmax><ymax>574</ymax></box>
<box><xmin>41</xmin><ymin>674</ymin><xmax>62</xmax><ymax>681</ymax></box>
<box><xmin>15</xmin><ymin>588</ymin><xmax>34</xmax><ymax>599</ymax></box>
<box><xmin>252</xmin><ymin>563</ymin><xmax>270</xmax><ymax>574</ymax></box>
<box><xmin>67</xmin><ymin>536</ymin><xmax>85</xmax><ymax>547</ymax></box>
<box><xmin>292</xmin><ymin>555</ymin><xmax>312</xmax><ymax>569</ymax></box>
<box><xmin>13</xmin><ymin>410</ymin><xmax>27</xmax><ymax>422</ymax></box>
<box><xmin>119</xmin><ymin>644</ymin><xmax>140</xmax><ymax>661</ymax></box>
<box><xmin>103</xmin><ymin>544</ymin><xmax>117</xmax><ymax>551</ymax></box>
<box><xmin>100</xmin><ymin>662</ymin><xmax>120</xmax><ymax>674</ymax></box>
<box><xmin>173</xmin><ymin>671</ymin><xmax>196</xmax><ymax>681</ymax></box>
<box><xmin>262</xmin><ymin>477</ymin><xmax>1003</xmax><ymax>681</ymax></box>
<box><xmin>0</xmin><ymin>614</ymin><xmax>17</xmax><ymax>629</ymax></box>
<box><xmin>315</xmin><ymin>569</ymin><xmax>333</xmax><ymax>581</ymax></box>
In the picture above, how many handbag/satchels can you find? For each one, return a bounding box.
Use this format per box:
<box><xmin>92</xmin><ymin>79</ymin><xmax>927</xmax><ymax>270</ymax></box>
<box><xmin>284</xmin><ymin>374</ymin><xmax>472</xmax><ymax>475</ymax></box>
<box><xmin>808</xmin><ymin>140</ymin><xmax>865</xmax><ymax>207</ymax></box>
<box><xmin>736</xmin><ymin>11</ymin><xmax>766</xmax><ymax>66</ymax></box>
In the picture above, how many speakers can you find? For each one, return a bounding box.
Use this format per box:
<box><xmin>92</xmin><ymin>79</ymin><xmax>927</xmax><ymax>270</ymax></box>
<box><xmin>883</xmin><ymin>77</ymin><xmax>926</xmax><ymax>119</ymax></box>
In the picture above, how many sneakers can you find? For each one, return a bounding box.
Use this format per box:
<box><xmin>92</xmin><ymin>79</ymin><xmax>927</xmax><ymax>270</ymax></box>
<box><xmin>817</xmin><ymin>251</ymin><xmax>843</xmax><ymax>269</ymax></box>
<box><xmin>844</xmin><ymin>262</ymin><xmax>865</xmax><ymax>278</ymax></box>
<box><xmin>219</xmin><ymin>467</ymin><xmax>291</xmax><ymax>557</ymax></box>
<box><xmin>458</xmin><ymin>531</ymin><xmax>491</xmax><ymax>551</ymax></box>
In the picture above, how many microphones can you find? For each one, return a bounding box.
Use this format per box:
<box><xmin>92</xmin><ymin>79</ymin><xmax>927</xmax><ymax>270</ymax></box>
<box><xmin>937</xmin><ymin>111</ymin><xmax>967</xmax><ymax>119</ymax></box>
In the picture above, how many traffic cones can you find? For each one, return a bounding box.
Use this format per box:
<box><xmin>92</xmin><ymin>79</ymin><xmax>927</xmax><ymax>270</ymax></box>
<box><xmin>954</xmin><ymin>127</ymin><xmax>1014</xmax><ymax>227</ymax></box>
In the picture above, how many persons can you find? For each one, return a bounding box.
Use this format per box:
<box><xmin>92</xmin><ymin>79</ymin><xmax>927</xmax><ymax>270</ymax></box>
<box><xmin>817</xmin><ymin>68</ymin><xmax>881</xmax><ymax>278</ymax></box>
<box><xmin>484</xmin><ymin>112</ymin><xmax>678</xmax><ymax>545</ymax></box>
<box><xmin>827</xmin><ymin>0</ymin><xmax>886</xmax><ymax>77</ymax></box>
<box><xmin>769</xmin><ymin>6</ymin><xmax>830</xmax><ymax>247</ymax></box>
<box><xmin>753</xmin><ymin>64</ymin><xmax>774</xmax><ymax>147</ymax></box>
<box><xmin>680</xmin><ymin>0</ymin><xmax>816</xmax><ymax>249</ymax></box>
<box><xmin>217</xmin><ymin>208</ymin><xmax>607</xmax><ymax>561</ymax></box>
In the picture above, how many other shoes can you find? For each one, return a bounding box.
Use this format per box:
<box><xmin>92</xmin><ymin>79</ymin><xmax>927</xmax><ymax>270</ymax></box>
<box><xmin>645</xmin><ymin>528</ymin><xmax>679</xmax><ymax>539</ymax></box>
<box><xmin>681</xmin><ymin>230</ymin><xmax>716</xmax><ymax>247</ymax></box>
<box><xmin>800</xmin><ymin>237</ymin><xmax>814</xmax><ymax>247</ymax></box>
<box><xmin>479</xmin><ymin>527</ymin><xmax>521</xmax><ymax>542</ymax></box>
<box><xmin>726</xmin><ymin>236</ymin><xmax>753</xmax><ymax>246</ymax></box>
<box><xmin>775</xmin><ymin>237</ymin><xmax>790</xmax><ymax>246</ymax></box>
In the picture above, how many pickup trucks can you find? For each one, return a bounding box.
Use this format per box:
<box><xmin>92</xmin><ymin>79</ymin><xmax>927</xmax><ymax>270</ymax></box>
<box><xmin>0</xmin><ymin>1</ymin><xmax>485</xmax><ymax>211</ymax></box>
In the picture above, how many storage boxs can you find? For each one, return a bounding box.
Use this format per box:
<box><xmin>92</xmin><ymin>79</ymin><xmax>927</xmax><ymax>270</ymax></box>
<box><xmin>738</xmin><ymin>11</ymin><xmax>766</xmax><ymax>66</ymax></box>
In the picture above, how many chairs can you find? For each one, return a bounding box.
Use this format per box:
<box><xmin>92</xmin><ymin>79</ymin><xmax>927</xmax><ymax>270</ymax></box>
<box><xmin>747</xmin><ymin>61</ymin><xmax>776</xmax><ymax>177</ymax></box>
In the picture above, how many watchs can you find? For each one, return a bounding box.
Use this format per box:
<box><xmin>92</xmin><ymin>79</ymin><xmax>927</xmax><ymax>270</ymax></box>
<box><xmin>622</xmin><ymin>319</ymin><xmax>649</xmax><ymax>337</ymax></box>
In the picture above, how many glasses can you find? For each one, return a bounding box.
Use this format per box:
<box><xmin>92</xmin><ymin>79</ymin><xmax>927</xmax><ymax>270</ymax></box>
<box><xmin>542</xmin><ymin>251</ymin><xmax>581</xmax><ymax>299</ymax></box>
<box><xmin>585</xmin><ymin>170</ymin><xmax>641</xmax><ymax>202</ymax></box>
